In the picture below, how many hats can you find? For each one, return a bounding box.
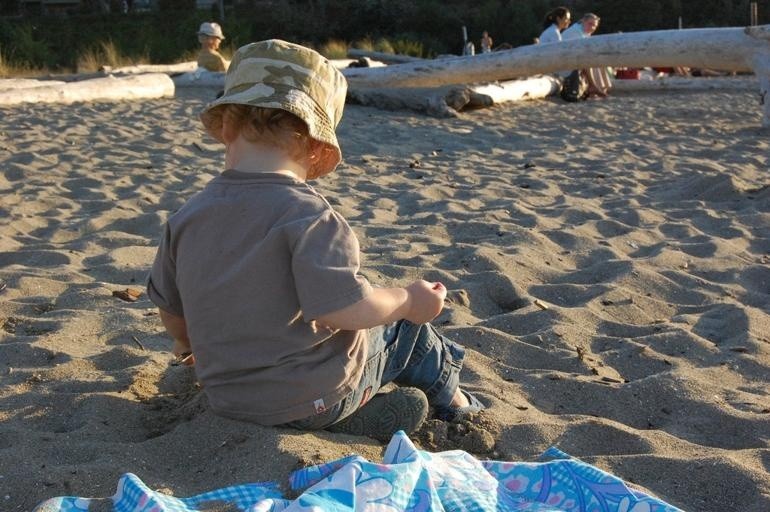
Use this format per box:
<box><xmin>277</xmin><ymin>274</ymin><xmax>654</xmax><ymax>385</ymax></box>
<box><xmin>198</xmin><ymin>39</ymin><xmax>347</xmax><ymax>181</ymax></box>
<box><xmin>195</xmin><ymin>23</ymin><xmax>224</xmax><ymax>41</ymax></box>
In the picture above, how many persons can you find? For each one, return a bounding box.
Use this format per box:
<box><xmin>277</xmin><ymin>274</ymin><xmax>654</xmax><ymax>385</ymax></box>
<box><xmin>148</xmin><ymin>38</ymin><xmax>490</xmax><ymax>444</ymax></box>
<box><xmin>196</xmin><ymin>22</ymin><xmax>228</xmax><ymax>74</ymax></box>
<box><xmin>458</xmin><ymin>8</ymin><xmax>739</xmax><ymax>101</ymax></box>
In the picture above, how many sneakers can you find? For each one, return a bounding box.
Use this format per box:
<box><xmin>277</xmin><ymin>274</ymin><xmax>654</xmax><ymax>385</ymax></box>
<box><xmin>433</xmin><ymin>387</ymin><xmax>487</xmax><ymax>426</ymax></box>
<box><xmin>322</xmin><ymin>388</ymin><xmax>429</xmax><ymax>442</ymax></box>
